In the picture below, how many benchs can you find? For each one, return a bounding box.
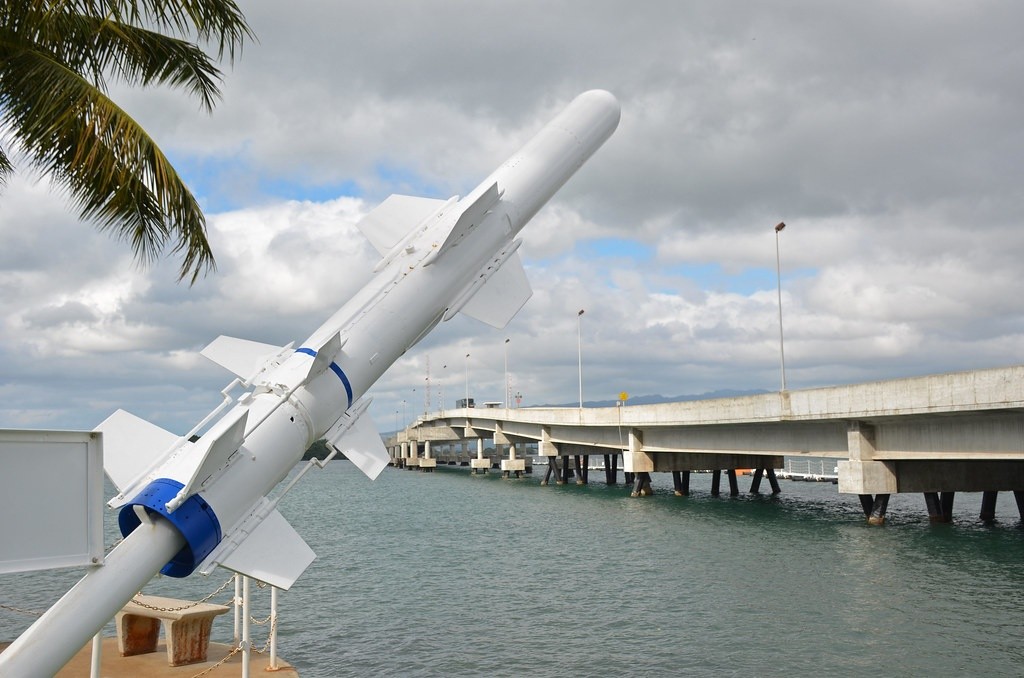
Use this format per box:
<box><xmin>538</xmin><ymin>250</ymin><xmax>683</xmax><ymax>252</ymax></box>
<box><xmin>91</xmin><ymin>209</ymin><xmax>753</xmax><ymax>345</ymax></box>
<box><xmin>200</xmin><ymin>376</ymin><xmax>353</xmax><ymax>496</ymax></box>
<box><xmin>115</xmin><ymin>595</ymin><xmax>231</xmax><ymax>667</ymax></box>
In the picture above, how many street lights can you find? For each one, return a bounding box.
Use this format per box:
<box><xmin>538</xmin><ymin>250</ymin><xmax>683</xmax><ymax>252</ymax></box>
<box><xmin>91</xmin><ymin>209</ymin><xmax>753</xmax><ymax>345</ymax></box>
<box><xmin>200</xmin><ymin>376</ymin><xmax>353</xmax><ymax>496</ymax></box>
<box><xmin>395</xmin><ymin>376</ymin><xmax>430</xmax><ymax>434</ymax></box>
<box><xmin>578</xmin><ymin>310</ymin><xmax>584</xmax><ymax>408</ymax></box>
<box><xmin>442</xmin><ymin>365</ymin><xmax>447</xmax><ymax>409</ymax></box>
<box><xmin>505</xmin><ymin>339</ymin><xmax>510</xmax><ymax>408</ymax></box>
<box><xmin>775</xmin><ymin>222</ymin><xmax>786</xmax><ymax>391</ymax></box>
<box><xmin>466</xmin><ymin>354</ymin><xmax>470</xmax><ymax>408</ymax></box>
